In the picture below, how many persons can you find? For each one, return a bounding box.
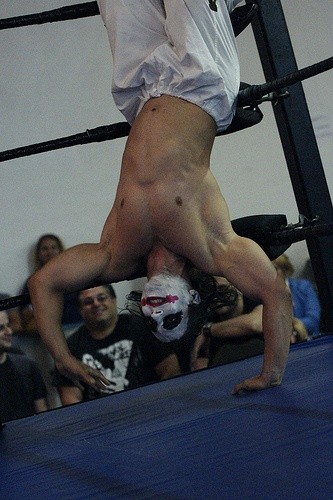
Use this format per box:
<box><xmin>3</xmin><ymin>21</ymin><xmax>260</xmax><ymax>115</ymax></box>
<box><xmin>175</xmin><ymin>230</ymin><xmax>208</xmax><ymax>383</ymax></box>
<box><xmin>24</xmin><ymin>0</ymin><xmax>296</xmax><ymax>395</ymax></box>
<box><xmin>49</xmin><ymin>282</ymin><xmax>183</xmax><ymax>408</ymax></box>
<box><xmin>0</xmin><ymin>221</ymin><xmax>333</xmax><ymax>423</ymax></box>
